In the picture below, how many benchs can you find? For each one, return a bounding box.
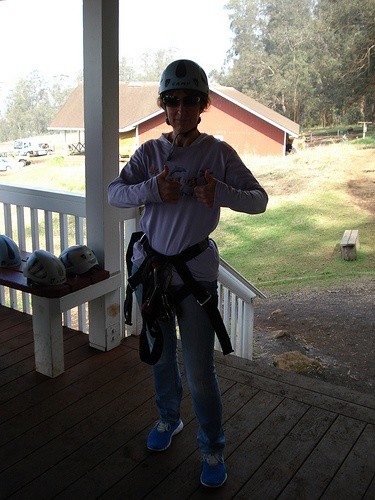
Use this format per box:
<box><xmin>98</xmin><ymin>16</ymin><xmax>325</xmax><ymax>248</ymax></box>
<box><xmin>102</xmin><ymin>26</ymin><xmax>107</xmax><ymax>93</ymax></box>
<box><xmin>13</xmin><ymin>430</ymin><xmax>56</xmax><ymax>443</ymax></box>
<box><xmin>340</xmin><ymin>230</ymin><xmax>360</xmax><ymax>260</ymax></box>
<box><xmin>0</xmin><ymin>250</ymin><xmax>122</xmax><ymax>377</ymax></box>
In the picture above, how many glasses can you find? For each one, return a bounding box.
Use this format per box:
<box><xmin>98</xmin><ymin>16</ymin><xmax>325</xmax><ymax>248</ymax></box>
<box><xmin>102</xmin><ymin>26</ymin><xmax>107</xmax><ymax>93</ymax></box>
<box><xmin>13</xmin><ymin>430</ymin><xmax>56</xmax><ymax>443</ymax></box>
<box><xmin>161</xmin><ymin>94</ymin><xmax>200</xmax><ymax>107</ymax></box>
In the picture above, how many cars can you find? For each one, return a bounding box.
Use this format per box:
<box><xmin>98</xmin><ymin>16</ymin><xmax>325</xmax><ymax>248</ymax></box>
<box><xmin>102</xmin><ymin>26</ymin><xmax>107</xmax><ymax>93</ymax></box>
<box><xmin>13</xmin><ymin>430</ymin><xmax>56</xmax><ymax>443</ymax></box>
<box><xmin>0</xmin><ymin>139</ymin><xmax>54</xmax><ymax>173</ymax></box>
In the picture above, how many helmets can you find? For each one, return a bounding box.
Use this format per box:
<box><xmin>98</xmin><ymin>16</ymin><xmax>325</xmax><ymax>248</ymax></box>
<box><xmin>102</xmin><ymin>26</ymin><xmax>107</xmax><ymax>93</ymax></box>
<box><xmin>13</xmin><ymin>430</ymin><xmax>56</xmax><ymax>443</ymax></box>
<box><xmin>23</xmin><ymin>249</ymin><xmax>67</xmax><ymax>288</ymax></box>
<box><xmin>59</xmin><ymin>245</ymin><xmax>99</xmax><ymax>278</ymax></box>
<box><xmin>158</xmin><ymin>60</ymin><xmax>209</xmax><ymax>95</ymax></box>
<box><xmin>0</xmin><ymin>235</ymin><xmax>21</xmax><ymax>268</ymax></box>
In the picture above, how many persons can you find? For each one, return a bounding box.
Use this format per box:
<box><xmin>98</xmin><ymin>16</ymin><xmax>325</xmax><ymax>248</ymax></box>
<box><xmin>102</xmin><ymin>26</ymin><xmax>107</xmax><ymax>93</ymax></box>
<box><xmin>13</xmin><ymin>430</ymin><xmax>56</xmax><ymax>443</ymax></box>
<box><xmin>107</xmin><ymin>59</ymin><xmax>269</xmax><ymax>487</ymax></box>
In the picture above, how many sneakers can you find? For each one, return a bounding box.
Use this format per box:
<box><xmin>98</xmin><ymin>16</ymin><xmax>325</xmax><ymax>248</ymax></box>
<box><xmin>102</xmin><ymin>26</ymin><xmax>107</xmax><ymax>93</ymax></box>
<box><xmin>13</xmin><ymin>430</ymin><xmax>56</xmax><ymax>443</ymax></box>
<box><xmin>146</xmin><ymin>418</ymin><xmax>183</xmax><ymax>451</ymax></box>
<box><xmin>200</xmin><ymin>452</ymin><xmax>227</xmax><ymax>488</ymax></box>
